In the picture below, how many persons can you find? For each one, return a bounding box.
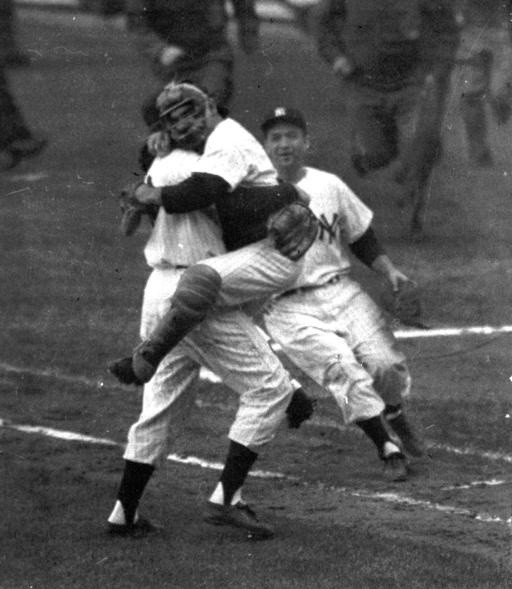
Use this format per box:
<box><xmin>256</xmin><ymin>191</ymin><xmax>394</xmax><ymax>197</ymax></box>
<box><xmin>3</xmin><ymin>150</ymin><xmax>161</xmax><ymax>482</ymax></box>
<box><xmin>1</xmin><ymin>0</ymin><xmax>52</xmax><ymax>176</ymax></box>
<box><xmin>95</xmin><ymin>0</ymin><xmax>265</xmax><ymax>129</ymax></box>
<box><xmin>105</xmin><ymin>75</ymin><xmax>319</xmax><ymax>541</ymax></box>
<box><xmin>307</xmin><ymin>0</ymin><xmax>459</xmax><ymax>238</ymax></box>
<box><xmin>454</xmin><ymin>0</ymin><xmax>510</xmax><ymax>167</ymax></box>
<box><xmin>254</xmin><ymin>106</ymin><xmax>428</xmax><ymax>484</ymax></box>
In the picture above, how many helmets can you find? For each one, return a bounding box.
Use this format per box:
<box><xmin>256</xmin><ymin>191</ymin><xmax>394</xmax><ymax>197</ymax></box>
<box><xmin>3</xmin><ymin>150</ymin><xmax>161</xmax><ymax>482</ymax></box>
<box><xmin>156</xmin><ymin>83</ymin><xmax>220</xmax><ymax>148</ymax></box>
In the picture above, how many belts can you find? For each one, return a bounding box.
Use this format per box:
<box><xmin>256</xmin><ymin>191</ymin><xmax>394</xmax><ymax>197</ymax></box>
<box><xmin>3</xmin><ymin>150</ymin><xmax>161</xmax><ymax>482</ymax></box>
<box><xmin>283</xmin><ymin>277</ymin><xmax>340</xmax><ymax>297</ymax></box>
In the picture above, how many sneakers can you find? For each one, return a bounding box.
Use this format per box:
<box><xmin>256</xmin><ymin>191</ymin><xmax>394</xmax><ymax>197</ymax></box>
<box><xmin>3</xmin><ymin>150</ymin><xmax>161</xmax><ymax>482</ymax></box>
<box><xmin>201</xmin><ymin>499</ymin><xmax>276</xmax><ymax>537</ymax></box>
<box><xmin>105</xmin><ymin>499</ymin><xmax>165</xmax><ymax>538</ymax></box>
<box><xmin>388</xmin><ymin>413</ymin><xmax>426</xmax><ymax>457</ymax></box>
<box><xmin>379</xmin><ymin>443</ymin><xmax>409</xmax><ymax>482</ymax></box>
<box><xmin>104</xmin><ymin>351</ymin><xmax>153</xmax><ymax>386</ymax></box>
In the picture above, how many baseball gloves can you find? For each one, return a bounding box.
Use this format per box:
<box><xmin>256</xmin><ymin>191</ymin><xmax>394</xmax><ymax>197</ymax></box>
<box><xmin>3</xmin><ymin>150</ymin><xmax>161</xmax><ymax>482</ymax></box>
<box><xmin>120</xmin><ymin>181</ymin><xmax>150</xmax><ymax>236</ymax></box>
<box><xmin>268</xmin><ymin>202</ymin><xmax>319</xmax><ymax>259</ymax></box>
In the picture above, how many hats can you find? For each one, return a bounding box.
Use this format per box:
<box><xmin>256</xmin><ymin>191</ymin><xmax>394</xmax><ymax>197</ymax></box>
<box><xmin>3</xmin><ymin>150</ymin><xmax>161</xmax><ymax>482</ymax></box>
<box><xmin>260</xmin><ymin>107</ymin><xmax>308</xmax><ymax>132</ymax></box>
